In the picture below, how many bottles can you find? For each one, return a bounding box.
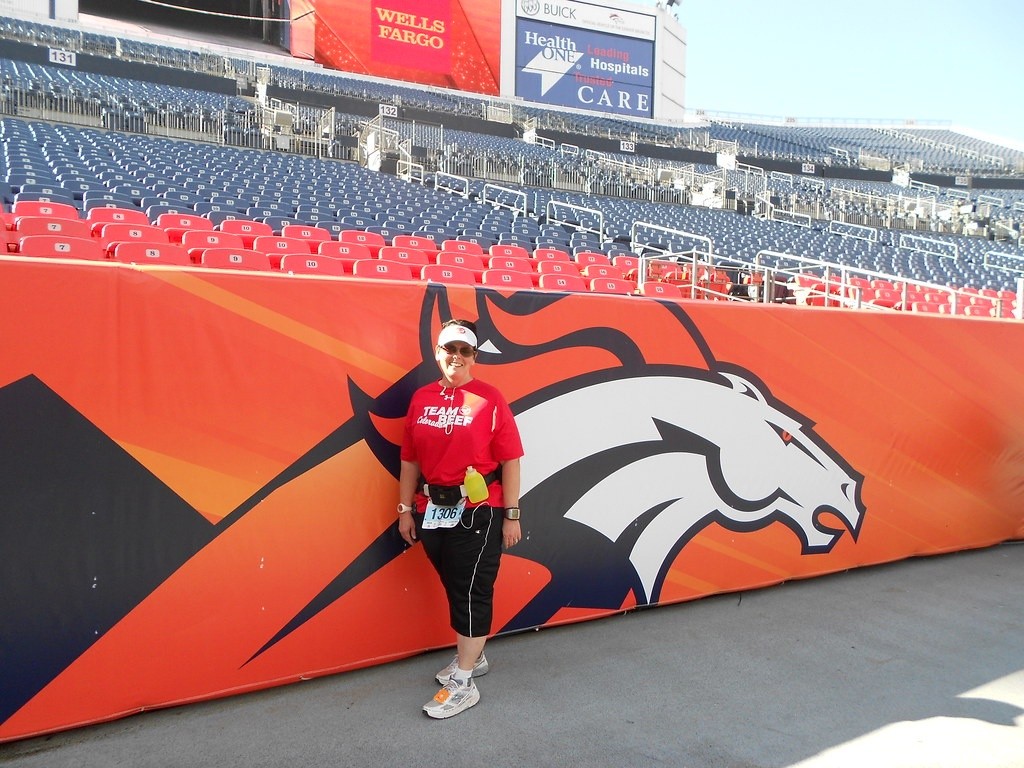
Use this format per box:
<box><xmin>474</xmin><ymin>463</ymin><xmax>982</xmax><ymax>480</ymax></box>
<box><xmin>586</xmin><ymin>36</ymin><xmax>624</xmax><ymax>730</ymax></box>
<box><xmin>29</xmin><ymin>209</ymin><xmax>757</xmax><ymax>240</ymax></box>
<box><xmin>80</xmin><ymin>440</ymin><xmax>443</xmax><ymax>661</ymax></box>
<box><xmin>464</xmin><ymin>465</ymin><xmax>489</xmax><ymax>504</ymax></box>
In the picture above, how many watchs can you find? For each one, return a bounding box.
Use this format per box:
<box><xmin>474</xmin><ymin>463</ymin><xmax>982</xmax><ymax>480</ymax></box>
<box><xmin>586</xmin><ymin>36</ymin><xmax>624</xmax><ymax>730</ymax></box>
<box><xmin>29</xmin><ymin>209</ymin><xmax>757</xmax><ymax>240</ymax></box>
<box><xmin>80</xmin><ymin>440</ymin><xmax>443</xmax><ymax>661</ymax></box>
<box><xmin>504</xmin><ymin>507</ymin><xmax>521</xmax><ymax>520</ymax></box>
<box><xmin>397</xmin><ymin>503</ymin><xmax>412</xmax><ymax>513</ymax></box>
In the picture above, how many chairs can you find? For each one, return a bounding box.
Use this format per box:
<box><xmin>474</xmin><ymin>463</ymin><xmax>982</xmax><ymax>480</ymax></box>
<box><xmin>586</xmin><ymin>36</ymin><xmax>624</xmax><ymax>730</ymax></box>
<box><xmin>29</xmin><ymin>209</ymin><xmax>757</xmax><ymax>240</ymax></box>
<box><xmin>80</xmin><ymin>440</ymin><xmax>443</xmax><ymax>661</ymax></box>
<box><xmin>0</xmin><ymin>15</ymin><xmax>1024</xmax><ymax>323</ymax></box>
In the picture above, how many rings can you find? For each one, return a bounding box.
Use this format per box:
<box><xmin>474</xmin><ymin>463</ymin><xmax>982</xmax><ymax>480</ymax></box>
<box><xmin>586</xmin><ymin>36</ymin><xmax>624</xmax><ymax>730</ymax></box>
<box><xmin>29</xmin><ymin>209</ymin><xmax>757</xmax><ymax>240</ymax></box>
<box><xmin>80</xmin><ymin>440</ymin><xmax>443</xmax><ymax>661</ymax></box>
<box><xmin>513</xmin><ymin>539</ymin><xmax>518</xmax><ymax>544</ymax></box>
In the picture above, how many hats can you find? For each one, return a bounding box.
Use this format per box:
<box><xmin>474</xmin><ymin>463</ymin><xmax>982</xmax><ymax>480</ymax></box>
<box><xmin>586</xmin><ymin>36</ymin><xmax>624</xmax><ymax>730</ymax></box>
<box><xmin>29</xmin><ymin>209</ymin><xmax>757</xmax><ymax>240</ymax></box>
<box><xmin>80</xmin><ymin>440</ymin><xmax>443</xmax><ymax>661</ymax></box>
<box><xmin>438</xmin><ymin>325</ymin><xmax>478</xmax><ymax>350</ymax></box>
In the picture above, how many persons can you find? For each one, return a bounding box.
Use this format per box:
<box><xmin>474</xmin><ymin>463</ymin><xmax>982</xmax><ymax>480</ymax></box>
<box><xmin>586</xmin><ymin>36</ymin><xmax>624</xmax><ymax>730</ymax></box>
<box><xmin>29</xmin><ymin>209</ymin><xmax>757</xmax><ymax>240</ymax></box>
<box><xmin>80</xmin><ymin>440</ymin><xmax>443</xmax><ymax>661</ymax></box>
<box><xmin>397</xmin><ymin>320</ymin><xmax>524</xmax><ymax>719</ymax></box>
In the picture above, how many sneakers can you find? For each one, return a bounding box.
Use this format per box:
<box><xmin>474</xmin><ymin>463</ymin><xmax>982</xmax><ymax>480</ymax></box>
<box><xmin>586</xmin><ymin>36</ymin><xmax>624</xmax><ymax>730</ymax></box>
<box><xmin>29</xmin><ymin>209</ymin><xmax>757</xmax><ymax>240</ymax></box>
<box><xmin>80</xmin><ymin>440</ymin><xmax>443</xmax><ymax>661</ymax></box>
<box><xmin>436</xmin><ymin>650</ymin><xmax>489</xmax><ymax>685</ymax></box>
<box><xmin>421</xmin><ymin>674</ymin><xmax>481</xmax><ymax>720</ymax></box>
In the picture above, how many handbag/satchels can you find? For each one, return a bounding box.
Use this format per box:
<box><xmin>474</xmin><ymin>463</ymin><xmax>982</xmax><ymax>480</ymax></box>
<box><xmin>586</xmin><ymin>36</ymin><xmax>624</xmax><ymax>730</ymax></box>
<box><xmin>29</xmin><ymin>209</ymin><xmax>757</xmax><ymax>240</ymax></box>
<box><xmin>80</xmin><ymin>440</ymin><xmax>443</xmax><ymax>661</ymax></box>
<box><xmin>417</xmin><ymin>469</ymin><xmax>499</xmax><ymax>507</ymax></box>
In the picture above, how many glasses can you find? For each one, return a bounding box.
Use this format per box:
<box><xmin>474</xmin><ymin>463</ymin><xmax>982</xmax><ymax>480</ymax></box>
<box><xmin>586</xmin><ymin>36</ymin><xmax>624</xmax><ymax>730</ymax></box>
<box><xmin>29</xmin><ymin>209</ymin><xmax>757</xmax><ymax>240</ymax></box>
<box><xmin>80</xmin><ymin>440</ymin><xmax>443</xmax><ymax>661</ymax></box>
<box><xmin>437</xmin><ymin>344</ymin><xmax>477</xmax><ymax>362</ymax></box>
